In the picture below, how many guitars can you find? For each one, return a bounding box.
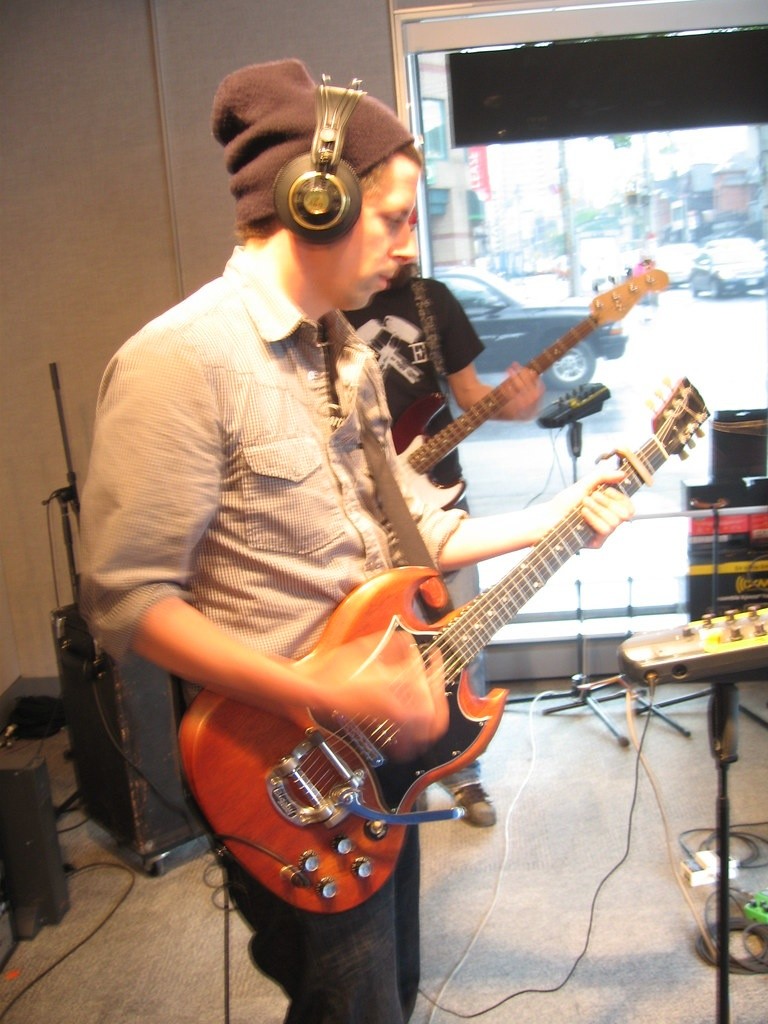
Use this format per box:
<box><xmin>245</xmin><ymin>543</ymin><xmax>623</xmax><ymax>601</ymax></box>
<box><xmin>176</xmin><ymin>375</ymin><xmax>712</xmax><ymax>917</ymax></box>
<box><xmin>348</xmin><ymin>264</ymin><xmax>670</xmax><ymax>512</ymax></box>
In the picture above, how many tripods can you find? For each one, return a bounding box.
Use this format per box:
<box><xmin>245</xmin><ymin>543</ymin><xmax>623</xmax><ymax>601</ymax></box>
<box><xmin>504</xmin><ymin>423</ymin><xmax>768</xmax><ymax>748</ymax></box>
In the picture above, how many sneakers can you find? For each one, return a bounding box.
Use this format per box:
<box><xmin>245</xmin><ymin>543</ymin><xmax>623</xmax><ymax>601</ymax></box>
<box><xmin>456</xmin><ymin>785</ymin><xmax>497</xmax><ymax>828</ymax></box>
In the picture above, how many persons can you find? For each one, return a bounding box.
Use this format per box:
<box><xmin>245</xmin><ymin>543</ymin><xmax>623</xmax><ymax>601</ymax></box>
<box><xmin>81</xmin><ymin>59</ymin><xmax>633</xmax><ymax>1024</ymax></box>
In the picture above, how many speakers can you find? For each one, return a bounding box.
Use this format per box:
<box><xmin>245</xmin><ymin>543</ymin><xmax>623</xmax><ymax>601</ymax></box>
<box><xmin>50</xmin><ymin>604</ymin><xmax>213</xmax><ymax>857</ymax></box>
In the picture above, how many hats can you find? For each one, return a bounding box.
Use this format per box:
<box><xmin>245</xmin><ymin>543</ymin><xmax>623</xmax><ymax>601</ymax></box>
<box><xmin>210</xmin><ymin>58</ymin><xmax>414</xmax><ymax>230</ymax></box>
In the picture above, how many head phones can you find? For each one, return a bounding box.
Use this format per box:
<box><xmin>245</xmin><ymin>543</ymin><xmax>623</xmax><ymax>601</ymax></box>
<box><xmin>272</xmin><ymin>72</ymin><xmax>364</xmax><ymax>240</ymax></box>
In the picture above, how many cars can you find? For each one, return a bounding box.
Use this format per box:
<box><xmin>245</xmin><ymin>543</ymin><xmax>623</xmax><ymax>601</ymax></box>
<box><xmin>690</xmin><ymin>235</ymin><xmax>768</xmax><ymax>298</ymax></box>
<box><xmin>433</xmin><ymin>264</ymin><xmax>631</xmax><ymax>391</ymax></box>
